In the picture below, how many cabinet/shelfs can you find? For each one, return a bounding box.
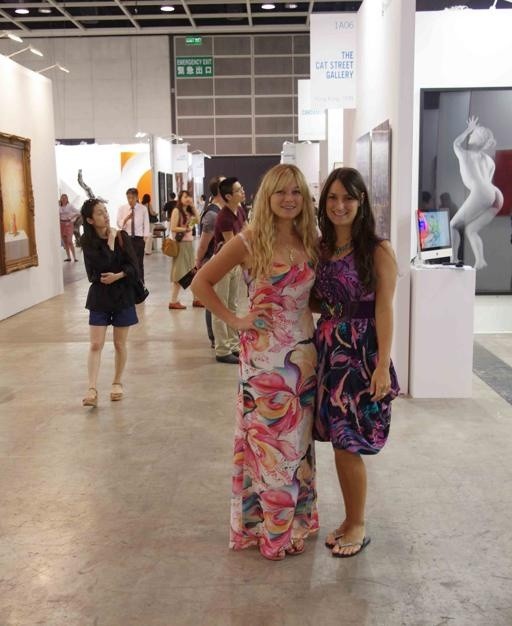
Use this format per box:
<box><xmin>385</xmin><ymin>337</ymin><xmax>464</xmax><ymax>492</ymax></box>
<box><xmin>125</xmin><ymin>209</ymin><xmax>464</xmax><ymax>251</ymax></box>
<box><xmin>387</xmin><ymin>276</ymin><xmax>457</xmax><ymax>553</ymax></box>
<box><xmin>408</xmin><ymin>265</ymin><xmax>476</xmax><ymax>398</ymax></box>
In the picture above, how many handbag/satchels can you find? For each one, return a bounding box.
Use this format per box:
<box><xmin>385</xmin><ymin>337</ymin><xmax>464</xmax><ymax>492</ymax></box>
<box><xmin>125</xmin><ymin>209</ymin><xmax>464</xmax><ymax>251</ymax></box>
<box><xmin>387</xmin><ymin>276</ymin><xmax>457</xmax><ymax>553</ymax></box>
<box><xmin>148</xmin><ymin>203</ymin><xmax>158</xmax><ymax>222</ymax></box>
<box><xmin>118</xmin><ymin>230</ymin><xmax>149</xmax><ymax>304</ymax></box>
<box><xmin>162</xmin><ymin>207</ymin><xmax>181</xmax><ymax>257</ymax></box>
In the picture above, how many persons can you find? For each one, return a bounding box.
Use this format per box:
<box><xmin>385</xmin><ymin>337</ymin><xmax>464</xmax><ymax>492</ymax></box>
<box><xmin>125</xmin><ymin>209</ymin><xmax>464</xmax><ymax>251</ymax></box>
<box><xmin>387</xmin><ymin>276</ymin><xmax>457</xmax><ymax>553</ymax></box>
<box><xmin>197</xmin><ymin>194</ymin><xmax>207</xmax><ymax>236</ymax></box>
<box><xmin>439</xmin><ymin>192</ymin><xmax>464</xmax><ymax>267</ymax></box>
<box><xmin>312</xmin><ymin>167</ymin><xmax>400</xmax><ymax>557</ymax></box>
<box><xmin>141</xmin><ymin>193</ymin><xmax>158</xmax><ymax>255</ymax></box>
<box><xmin>191</xmin><ymin>164</ymin><xmax>322</xmax><ymax>560</ymax></box>
<box><xmin>186</xmin><ymin>225</ymin><xmax>190</xmax><ymax>232</ymax></box>
<box><xmin>210</xmin><ymin>176</ymin><xmax>247</xmax><ymax>363</ymax></box>
<box><xmin>78</xmin><ymin>198</ymin><xmax>137</xmax><ymax>408</ymax></box>
<box><xmin>59</xmin><ymin>194</ymin><xmax>80</xmax><ymax>262</ymax></box>
<box><xmin>194</xmin><ymin>176</ymin><xmax>227</xmax><ymax>349</ymax></box>
<box><xmin>160</xmin><ymin>193</ymin><xmax>178</xmax><ymax>236</ymax></box>
<box><xmin>416</xmin><ymin>189</ymin><xmax>434</xmax><ymax>210</ymax></box>
<box><xmin>169</xmin><ymin>190</ymin><xmax>204</xmax><ymax>309</ymax></box>
<box><xmin>117</xmin><ymin>189</ymin><xmax>150</xmax><ymax>287</ymax></box>
<box><xmin>444</xmin><ymin>115</ymin><xmax>505</xmax><ymax>271</ymax></box>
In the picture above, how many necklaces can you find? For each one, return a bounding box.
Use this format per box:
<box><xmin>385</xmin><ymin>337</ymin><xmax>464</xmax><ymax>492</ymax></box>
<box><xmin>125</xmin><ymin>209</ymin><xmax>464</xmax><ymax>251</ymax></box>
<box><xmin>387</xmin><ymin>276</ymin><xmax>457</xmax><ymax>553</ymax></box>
<box><xmin>328</xmin><ymin>230</ymin><xmax>353</xmax><ymax>256</ymax></box>
<box><xmin>271</xmin><ymin>224</ymin><xmax>301</xmax><ymax>260</ymax></box>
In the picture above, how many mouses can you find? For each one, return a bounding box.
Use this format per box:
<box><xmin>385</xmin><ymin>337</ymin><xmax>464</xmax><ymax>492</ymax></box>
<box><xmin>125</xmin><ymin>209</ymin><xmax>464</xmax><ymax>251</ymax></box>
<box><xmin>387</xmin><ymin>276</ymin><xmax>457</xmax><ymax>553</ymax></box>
<box><xmin>456</xmin><ymin>260</ymin><xmax>464</xmax><ymax>267</ymax></box>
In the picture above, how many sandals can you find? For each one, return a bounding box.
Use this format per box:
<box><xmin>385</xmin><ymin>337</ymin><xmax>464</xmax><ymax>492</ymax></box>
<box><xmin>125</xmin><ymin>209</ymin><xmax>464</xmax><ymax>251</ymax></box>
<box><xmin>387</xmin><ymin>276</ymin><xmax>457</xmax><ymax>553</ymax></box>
<box><xmin>110</xmin><ymin>382</ymin><xmax>123</xmax><ymax>400</ymax></box>
<box><xmin>82</xmin><ymin>388</ymin><xmax>97</xmax><ymax>406</ymax></box>
<box><xmin>288</xmin><ymin>540</ymin><xmax>305</xmax><ymax>555</ymax></box>
<box><xmin>259</xmin><ymin>543</ymin><xmax>285</xmax><ymax>560</ymax></box>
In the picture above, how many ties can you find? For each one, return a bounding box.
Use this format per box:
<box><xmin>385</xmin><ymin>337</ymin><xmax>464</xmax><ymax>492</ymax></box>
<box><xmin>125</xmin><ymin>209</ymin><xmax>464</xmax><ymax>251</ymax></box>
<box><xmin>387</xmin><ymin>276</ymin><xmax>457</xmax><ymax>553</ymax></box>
<box><xmin>130</xmin><ymin>207</ymin><xmax>135</xmax><ymax>239</ymax></box>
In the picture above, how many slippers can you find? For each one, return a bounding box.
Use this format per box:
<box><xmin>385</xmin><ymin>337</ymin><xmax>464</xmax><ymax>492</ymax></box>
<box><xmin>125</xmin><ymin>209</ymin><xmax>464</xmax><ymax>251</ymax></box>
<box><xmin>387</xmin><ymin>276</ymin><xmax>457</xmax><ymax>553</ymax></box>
<box><xmin>325</xmin><ymin>525</ymin><xmax>346</xmax><ymax>548</ymax></box>
<box><xmin>333</xmin><ymin>536</ymin><xmax>371</xmax><ymax>556</ymax></box>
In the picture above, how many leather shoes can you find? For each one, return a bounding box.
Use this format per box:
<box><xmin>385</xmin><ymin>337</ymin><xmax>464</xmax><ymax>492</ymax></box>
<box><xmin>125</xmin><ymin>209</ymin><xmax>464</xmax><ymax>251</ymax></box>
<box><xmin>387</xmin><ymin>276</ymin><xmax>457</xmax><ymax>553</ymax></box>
<box><xmin>216</xmin><ymin>353</ymin><xmax>240</xmax><ymax>364</ymax></box>
<box><xmin>64</xmin><ymin>258</ymin><xmax>72</xmax><ymax>261</ymax></box>
<box><xmin>169</xmin><ymin>301</ymin><xmax>187</xmax><ymax>309</ymax></box>
<box><xmin>74</xmin><ymin>257</ymin><xmax>79</xmax><ymax>261</ymax></box>
<box><xmin>193</xmin><ymin>300</ymin><xmax>206</xmax><ymax>307</ymax></box>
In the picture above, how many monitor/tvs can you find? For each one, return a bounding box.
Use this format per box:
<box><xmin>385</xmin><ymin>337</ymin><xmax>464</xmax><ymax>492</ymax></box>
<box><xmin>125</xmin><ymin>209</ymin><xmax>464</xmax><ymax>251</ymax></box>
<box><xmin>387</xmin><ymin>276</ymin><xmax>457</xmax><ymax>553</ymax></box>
<box><xmin>417</xmin><ymin>207</ymin><xmax>455</xmax><ymax>264</ymax></box>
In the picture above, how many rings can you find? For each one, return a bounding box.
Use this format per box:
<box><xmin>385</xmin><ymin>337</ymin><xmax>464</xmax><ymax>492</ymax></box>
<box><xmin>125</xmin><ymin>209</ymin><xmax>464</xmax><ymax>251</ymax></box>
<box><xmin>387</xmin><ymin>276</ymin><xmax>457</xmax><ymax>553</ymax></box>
<box><xmin>253</xmin><ymin>320</ymin><xmax>263</xmax><ymax>334</ymax></box>
<box><xmin>381</xmin><ymin>392</ymin><xmax>388</xmax><ymax>396</ymax></box>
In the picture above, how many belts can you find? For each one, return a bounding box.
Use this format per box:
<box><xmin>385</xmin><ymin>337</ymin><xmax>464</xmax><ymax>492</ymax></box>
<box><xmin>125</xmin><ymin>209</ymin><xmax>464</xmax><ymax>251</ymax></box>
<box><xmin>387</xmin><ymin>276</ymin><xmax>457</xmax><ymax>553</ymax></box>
<box><xmin>129</xmin><ymin>235</ymin><xmax>144</xmax><ymax>240</ymax></box>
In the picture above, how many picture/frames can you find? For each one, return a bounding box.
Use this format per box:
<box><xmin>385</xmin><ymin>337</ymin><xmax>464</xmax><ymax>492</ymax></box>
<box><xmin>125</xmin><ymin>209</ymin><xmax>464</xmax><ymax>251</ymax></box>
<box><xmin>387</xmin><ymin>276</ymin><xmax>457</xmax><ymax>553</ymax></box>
<box><xmin>0</xmin><ymin>132</ymin><xmax>38</xmax><ymax>275</ymax></box>
<box><xmin>188</xmin><ymin>177</ymin><xmax>194</xmax><ymax>203</ymax></box>
<box><xmin>175</xmin><ymin>172</ymin><xmax>183</xmax><ymax>197</ymax></box>
<box><xmin>355</xmin><ymin>132</ymin><xmax>370</xmax><ymax>207</ymax></box>
<box><xmin>166</xmin><ymin>174</ymin><xmax>173</xmax><ymax>221</ymax></box>
<box><xmin>492</xmin><ymin>149</ymin><xmax>512</xmax><ymax>216</ymax></box>
<box><xmin>371</xmin><ymin>119</ymin><xmax>391</xmax><ymax>240</ymax></box>
<box><xmin>159</xmin><ymin>172</ymin><xmax>166</xmax><ymax>222</ymax></box>
